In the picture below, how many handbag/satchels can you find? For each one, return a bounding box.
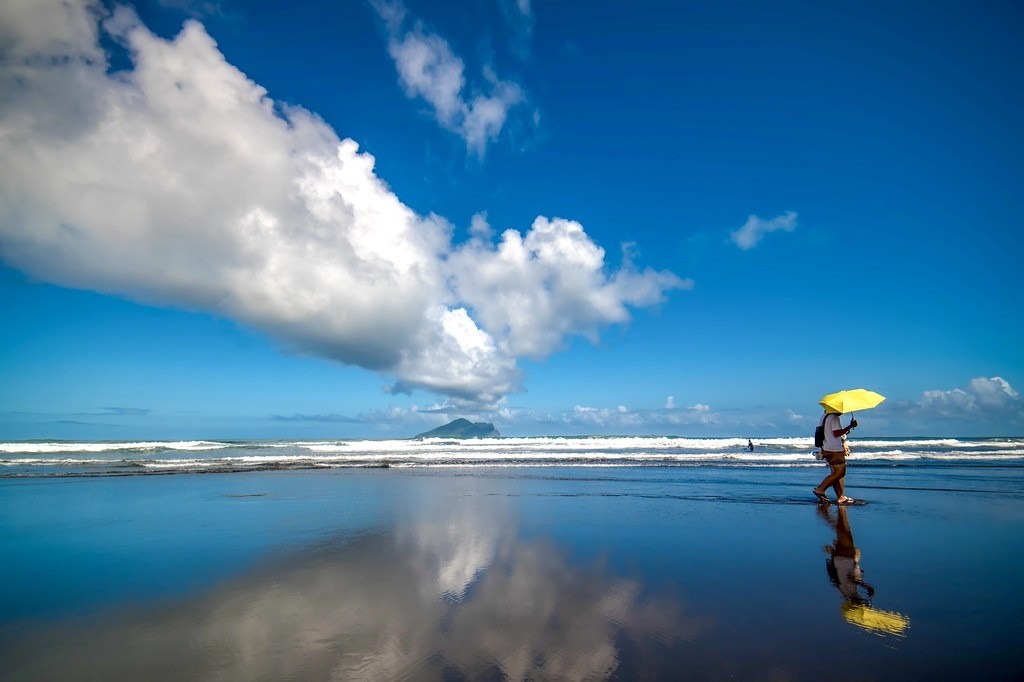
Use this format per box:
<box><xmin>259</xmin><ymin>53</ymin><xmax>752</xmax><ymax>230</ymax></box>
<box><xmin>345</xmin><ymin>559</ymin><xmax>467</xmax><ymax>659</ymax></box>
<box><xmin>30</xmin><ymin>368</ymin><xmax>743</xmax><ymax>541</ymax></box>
<box><xmin>814</xmin><ymin>424</ymin><xmax>826</xmax><ymax>447</ymax></box>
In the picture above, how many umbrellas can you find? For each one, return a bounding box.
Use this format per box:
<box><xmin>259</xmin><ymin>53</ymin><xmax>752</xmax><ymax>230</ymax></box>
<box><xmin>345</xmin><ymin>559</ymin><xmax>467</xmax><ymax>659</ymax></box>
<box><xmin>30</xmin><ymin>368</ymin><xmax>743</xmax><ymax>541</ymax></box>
<box><xmin>839</xmin><ymin>591</ymin><xmax>911</xmax><ymax>639</ymax></box>
<box><xmin>818</xmin><ymin>388</ymin><xmax>886</xmax><ymax>421</ymax></box>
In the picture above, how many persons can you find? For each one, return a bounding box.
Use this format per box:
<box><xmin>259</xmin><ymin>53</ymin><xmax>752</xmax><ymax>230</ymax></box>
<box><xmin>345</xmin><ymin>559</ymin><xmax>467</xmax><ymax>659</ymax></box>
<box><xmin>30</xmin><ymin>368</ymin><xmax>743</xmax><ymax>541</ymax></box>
<box><xmin>816</xmin><ymin>504</ymin><xmax>875</xmax><ymax>605</ymax></box>
<box><xmin>744</xmin><ymin>438</ymin><xmax>754</xmax><ymax>452</ymax></box>
<box><xmin>811</xmin><ymin>409</ymin><xmax>858</xmax><ymax>505</ymax></box>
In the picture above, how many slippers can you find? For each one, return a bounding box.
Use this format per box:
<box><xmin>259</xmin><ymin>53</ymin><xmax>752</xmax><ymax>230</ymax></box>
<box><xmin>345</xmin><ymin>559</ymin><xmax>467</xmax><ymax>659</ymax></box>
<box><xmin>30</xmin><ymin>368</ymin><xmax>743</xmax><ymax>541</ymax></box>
<box><xmin>836</xmin><ymin>497</ymin><xmax>854</xmax><ymax>504</ymax></box>
<box><xmin>812</xmin><ymin>490</ymin><xmax>830</xmax><ymax>503</ymax></box>
